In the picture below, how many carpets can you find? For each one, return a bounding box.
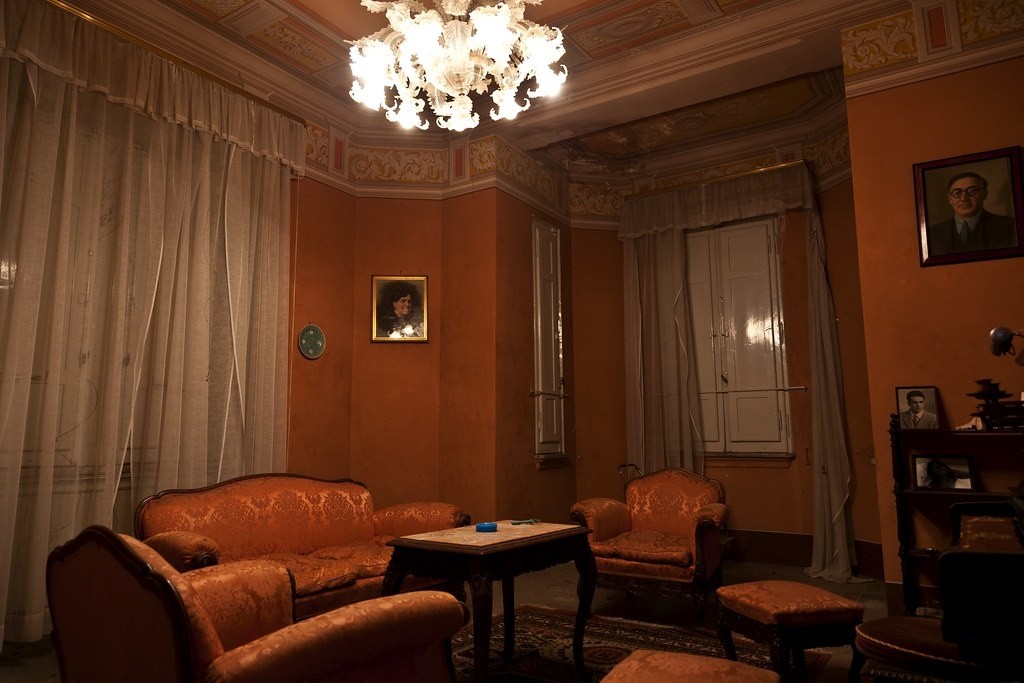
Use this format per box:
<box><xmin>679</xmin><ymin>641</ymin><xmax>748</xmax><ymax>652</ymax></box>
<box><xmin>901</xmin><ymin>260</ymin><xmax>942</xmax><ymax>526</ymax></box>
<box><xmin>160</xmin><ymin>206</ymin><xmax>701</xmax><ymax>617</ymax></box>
<box><xmin>450</xmin><ymin>599</ymin><xmax>834</xmax><ymax>682</ymax></box>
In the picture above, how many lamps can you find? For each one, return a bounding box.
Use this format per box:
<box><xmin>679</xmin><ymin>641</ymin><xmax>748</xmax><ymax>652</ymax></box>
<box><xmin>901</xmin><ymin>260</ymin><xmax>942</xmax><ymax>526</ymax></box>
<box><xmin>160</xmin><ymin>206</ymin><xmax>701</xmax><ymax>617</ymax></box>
<box><xmin>342</xmin><ymin>0</ymin><xmax>571</xmax><ymax>134</ymax></box>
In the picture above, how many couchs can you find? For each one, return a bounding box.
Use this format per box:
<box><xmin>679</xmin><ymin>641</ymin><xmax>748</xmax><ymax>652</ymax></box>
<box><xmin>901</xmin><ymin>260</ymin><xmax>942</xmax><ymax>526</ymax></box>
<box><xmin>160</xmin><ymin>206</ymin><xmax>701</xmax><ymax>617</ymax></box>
<box><xmin>42</xmin><ymin>522</ymin><xmax>472</xmax><ymax>682</ymax></box>
<box><xmin>570</xmin><ymin>465</ymin><xmax>730</xmax><ymax>631</ymax></box>
<box><xmin>135</xmin><ymin>471</ymin><xmax>473</xmax><ymax>626</ymax></box>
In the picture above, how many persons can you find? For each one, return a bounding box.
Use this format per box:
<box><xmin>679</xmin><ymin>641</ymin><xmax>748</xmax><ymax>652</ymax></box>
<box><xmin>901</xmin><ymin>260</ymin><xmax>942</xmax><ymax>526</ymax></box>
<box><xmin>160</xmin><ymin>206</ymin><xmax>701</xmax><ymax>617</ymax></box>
<box><xmin>921</xmin><ymin>155</ymin><xmax>1020</xmax><ymax>257</ymax></box>
<box><xmin>897</xmin><ymin>387</ymin><xmax>938</xmax><ymax>428</ymax></box>
<box><xmin>376</xmin><ymin>279</ymin><xmax>425</xmax><ymax>338</ymax></box>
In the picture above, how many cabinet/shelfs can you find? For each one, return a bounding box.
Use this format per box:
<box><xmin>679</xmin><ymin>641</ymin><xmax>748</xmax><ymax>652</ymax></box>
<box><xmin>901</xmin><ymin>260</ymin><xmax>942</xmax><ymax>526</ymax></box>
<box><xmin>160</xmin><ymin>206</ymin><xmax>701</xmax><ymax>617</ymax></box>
<box><xmin>887</xmin><ymin>380</ymin><xmax>1024</xmax><ymax>618</ymax></box>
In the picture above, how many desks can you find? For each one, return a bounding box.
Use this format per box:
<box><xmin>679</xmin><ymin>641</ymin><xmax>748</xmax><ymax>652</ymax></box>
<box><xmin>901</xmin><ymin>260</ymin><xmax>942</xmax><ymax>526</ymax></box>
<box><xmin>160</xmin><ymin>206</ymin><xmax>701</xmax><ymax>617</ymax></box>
<box><xmin>853</xmin><ymin>611</ymin><xmax>1024</xmax><ymax>683</ymax></box>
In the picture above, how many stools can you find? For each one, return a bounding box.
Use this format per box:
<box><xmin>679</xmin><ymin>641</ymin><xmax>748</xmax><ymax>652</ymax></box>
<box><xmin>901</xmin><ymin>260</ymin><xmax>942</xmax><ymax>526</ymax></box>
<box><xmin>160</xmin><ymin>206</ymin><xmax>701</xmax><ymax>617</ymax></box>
<box><xmin>598</xmin><ymin>648</ymin><xmax>784</xmax><ymax>682</ymax></box>
<box><xmin>714</xmin><ymin>578</ymin><xmax>868</xmax><ymax>683</ymax></box>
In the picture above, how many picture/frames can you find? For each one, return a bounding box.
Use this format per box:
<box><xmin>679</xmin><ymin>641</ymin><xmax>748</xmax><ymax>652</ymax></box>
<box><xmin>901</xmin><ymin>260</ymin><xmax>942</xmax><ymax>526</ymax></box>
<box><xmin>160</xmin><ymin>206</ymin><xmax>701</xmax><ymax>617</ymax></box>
<box><xmin>912</xmin><ymin>144</ymin><xmax>1024</xmax><ymax>267</ymax></box>
<box><xmin>895</xmin><ymin>385</ymin><xmax>941</xmax><ymax>431</ymax></box>
<box><xmin>907</xmin><ymin>452</ymin><xmax>978</xmax><ymax>494</ymax></box>
<box><xmin>370</xmin><ymin>273</ymin><xmax>429</xmax><ymax>343</ymax></box>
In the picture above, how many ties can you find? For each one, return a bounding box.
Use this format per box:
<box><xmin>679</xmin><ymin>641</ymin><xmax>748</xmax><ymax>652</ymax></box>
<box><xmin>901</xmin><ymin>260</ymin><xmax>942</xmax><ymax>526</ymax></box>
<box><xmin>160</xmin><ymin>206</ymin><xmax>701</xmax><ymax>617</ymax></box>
<box><xmin>912</xmin><ymin>415</ymin><xmax>918</xmax><ymax>428</ymax></box>
